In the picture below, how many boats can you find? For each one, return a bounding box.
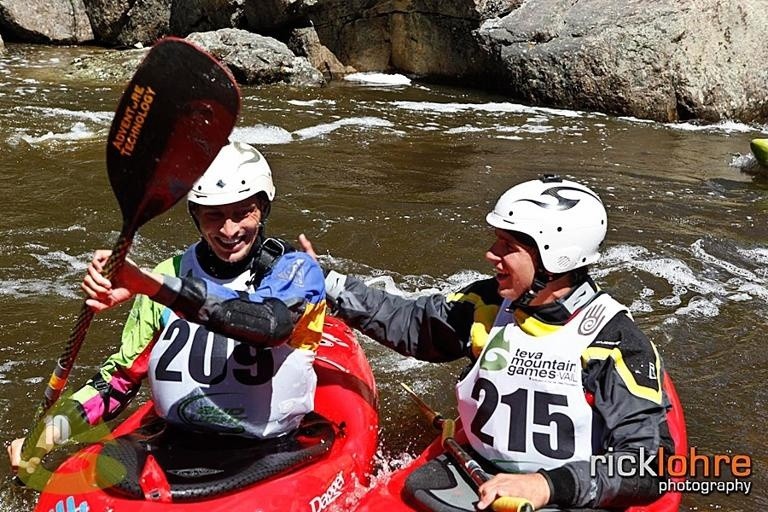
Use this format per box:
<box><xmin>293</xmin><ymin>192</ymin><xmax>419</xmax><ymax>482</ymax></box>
<box><xmin>30</xmin><ymin>306</ymin><xmax>381</xmax><ymax>512</ymax></box>
<box><xmin>348</xmin><ymin>367</ymin><xmax>692</xmax><ymax>512</ymax></box>
<box><xmin>749</xmin><ymin>136</ymin><xmax>768</xmax><ymax>171</ymax></box>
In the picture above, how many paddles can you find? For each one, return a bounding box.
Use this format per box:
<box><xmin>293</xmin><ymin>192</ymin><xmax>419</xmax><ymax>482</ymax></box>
<box><xmin>13</xmin><ymin>37</ymin><xmax>243</xmax><ymax>461</ymax></box>
<box><xmin>400</xmin><ymin>381</ymin><xmax>534</xmax><ymax>512</ymax></box>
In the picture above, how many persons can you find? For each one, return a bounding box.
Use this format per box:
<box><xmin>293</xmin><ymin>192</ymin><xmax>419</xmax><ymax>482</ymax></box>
<box><xmin>297</xmin><ymin>174</ymin><xmax>676</xmax><ymax>511</ymax></box>
<box><xmin>5</xmin><ymin>143</ymin><xmax>329</xmax><ymax>484</ymax></box>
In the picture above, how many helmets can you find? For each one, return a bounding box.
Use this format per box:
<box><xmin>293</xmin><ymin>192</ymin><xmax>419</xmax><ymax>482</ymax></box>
<box><xmin>485</xmin><ymin>178</ymin><xmax>609</xmax><ymax>275</ymax></box>
<box><xmin>186</xmin><ymin>140</ymin><xmax>276</xmax><ymax>236</ymax></box>
